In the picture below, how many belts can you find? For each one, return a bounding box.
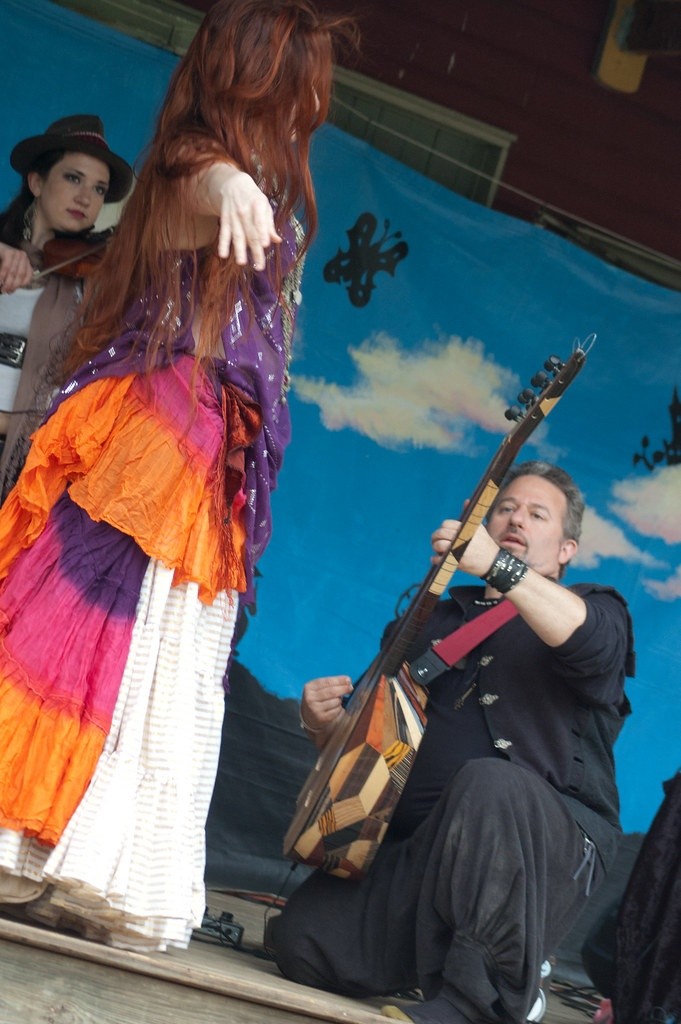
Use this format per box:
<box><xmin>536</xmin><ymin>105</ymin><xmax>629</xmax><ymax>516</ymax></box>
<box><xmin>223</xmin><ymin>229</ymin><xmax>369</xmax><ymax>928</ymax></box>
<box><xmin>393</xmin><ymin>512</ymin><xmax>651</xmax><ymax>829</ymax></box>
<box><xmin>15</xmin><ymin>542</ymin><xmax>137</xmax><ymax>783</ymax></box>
<box><xmin>0</xmin><ymin>333</ymin><xmax>27</xmax><ymax>370</ymax></box>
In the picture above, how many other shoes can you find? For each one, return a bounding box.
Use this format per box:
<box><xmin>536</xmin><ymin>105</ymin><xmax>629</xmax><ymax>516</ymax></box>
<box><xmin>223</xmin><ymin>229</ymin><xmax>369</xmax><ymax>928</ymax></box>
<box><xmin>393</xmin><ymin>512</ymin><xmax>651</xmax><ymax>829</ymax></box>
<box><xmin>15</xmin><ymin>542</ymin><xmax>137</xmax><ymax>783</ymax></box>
<box><xmin>379</xmin><ymin>995</ymin><xmax>472</xmax><ymax>1024</ymax></box>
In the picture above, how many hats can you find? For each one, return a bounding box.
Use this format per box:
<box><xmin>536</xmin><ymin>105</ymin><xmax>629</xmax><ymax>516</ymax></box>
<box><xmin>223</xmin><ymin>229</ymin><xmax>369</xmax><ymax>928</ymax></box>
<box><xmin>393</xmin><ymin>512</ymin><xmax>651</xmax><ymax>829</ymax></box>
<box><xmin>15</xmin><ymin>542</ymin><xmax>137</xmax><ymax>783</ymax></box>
<box><xmin>10</xmin><ymin>114</ymin><xmax>133</xmax><ymax>204</ymax></box>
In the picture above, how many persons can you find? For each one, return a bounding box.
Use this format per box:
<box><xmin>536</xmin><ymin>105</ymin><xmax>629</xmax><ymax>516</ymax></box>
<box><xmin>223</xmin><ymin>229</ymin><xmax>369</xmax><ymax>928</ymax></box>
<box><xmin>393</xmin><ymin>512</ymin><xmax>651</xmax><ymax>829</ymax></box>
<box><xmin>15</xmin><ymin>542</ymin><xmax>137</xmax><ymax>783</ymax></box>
<box><xmin>1</xmin><ymin>112</ymin><xmax>138</xmax><ymax>508</ymax></box>
<box><xmin>269</xmin><ymin>461</ymin><xmax>637</xmax><ymax>1024</ymax></box>
<box><xmin>0</xmin><ymin>1</ymin><xmax>363</xmax><ymax>952</ymax></box>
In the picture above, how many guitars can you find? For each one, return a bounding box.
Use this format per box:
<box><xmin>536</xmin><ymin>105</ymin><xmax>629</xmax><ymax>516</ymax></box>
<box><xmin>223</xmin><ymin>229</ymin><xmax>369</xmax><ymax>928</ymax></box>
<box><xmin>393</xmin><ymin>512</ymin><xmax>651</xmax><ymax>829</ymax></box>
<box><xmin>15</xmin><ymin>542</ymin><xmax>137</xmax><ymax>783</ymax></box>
<box><xmin>283</xmin><ymin>330</ymin><xmax>599</xmax><ymax>877</ymax></box>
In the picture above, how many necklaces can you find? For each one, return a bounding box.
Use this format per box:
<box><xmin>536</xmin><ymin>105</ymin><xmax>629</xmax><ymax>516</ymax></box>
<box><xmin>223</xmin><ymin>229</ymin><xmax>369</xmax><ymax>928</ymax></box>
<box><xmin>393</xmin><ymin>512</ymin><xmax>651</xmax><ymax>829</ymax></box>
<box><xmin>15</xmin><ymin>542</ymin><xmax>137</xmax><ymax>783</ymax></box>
<box><xmin>471</xmin><ymin>597</ymin><xmax>499</xmax><ymax>605</ymax></box>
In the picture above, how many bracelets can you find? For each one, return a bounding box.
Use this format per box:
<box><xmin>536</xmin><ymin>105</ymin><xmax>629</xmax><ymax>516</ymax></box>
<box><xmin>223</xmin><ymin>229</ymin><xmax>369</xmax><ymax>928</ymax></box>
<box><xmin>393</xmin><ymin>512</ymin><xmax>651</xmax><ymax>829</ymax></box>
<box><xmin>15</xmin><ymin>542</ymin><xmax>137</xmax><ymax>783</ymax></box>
<box><xmin>296</xmin><ymin>706</ymin><xmax>322</xmax><ymax>734</ymax></box>
<box><xmin>482</xmin><ymin>550</ymin><xmax>526</xmax><ymax>593</ymax></box>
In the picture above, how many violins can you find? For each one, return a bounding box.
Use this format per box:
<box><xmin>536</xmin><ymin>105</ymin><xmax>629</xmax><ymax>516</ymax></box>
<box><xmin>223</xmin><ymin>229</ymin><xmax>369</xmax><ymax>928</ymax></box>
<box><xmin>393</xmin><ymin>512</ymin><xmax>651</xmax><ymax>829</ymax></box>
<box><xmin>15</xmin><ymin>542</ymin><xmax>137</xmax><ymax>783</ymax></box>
<box><xmin>42</xmin><ymin>230</ymin><xmax>118</xmax><ymax>278</ymax></box>
